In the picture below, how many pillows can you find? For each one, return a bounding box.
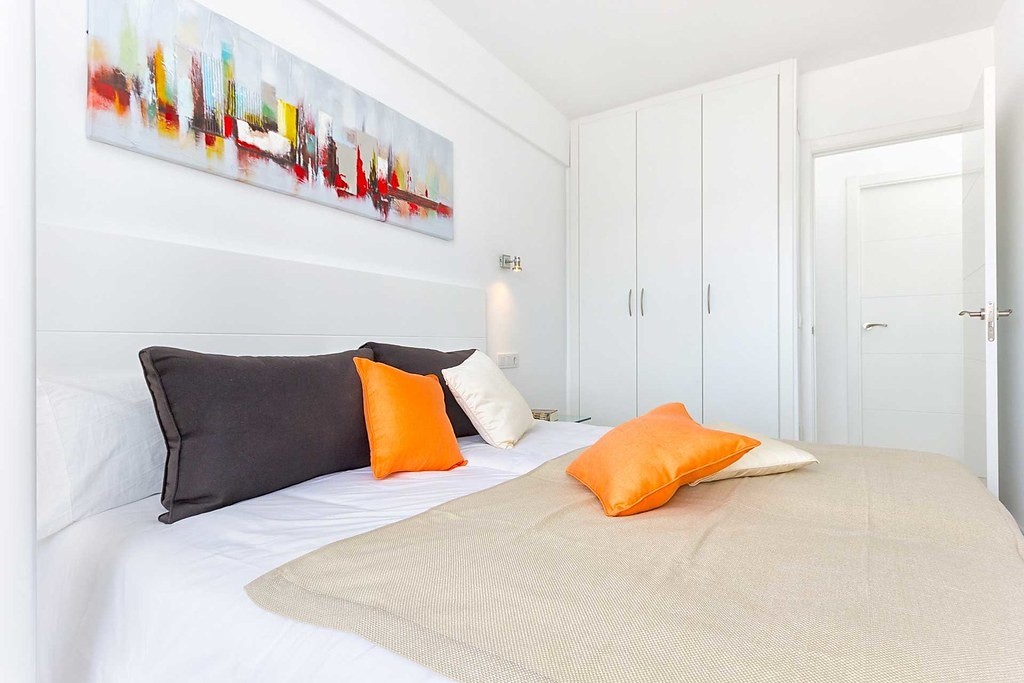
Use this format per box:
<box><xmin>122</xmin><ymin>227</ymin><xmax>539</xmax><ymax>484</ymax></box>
<box><xmin>138</xmin><ymin>346</ymin><xmax>374</xmax><ymax>524</ymax></box>
<box><xmin>688</xmin><ymin>423</ymin><xmax>819</xmax><ymax>487</ymax></box>
<box><xmin>352</xmin><ymin>356</ymin><xmax>468</xmax><ymax>479</ymax></box>
<box><xmin>565</xmin><ymin>402</ymin><xmax>762</xmax><ymax>517</ymax></box>
<box><xmin>361</xmin><ymin>343</ymin><xmax>481</xmax><ymax>438</ymax></box>
<box><xmin>441</xmin><ymin>349</ymin><xmax>535</xmax><ymax>450</ymax></box>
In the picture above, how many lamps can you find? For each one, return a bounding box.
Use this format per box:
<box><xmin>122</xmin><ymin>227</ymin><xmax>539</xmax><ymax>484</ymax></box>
<box><xmin>500</xmin><ymin>254</ymin><xmax>522</xmax><ymax>272</ymax></box>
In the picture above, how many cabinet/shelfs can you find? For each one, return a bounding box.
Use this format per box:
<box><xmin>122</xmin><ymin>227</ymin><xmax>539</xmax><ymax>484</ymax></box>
<box><xmin>702</xmin><ymin>59</ymin><xmax>801</xmax><ymax>444</ymax></box>
<box><xmin>569</xmin><ymin>85</ymin><xmax>700</xmax><ymax>431</ymax></box>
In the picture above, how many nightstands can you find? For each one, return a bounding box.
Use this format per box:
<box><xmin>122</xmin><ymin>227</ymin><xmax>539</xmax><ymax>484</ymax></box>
<box><xmin>558</xmin><ymin>414</ymin><xmax>591</xmax><ymax>423</ymax></box>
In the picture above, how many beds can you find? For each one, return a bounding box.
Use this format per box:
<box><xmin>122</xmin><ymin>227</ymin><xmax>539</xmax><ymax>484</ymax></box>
<box><xmin>36</xmin><ymin>233</ymin><xmax>1024</xmax><ymax>683</ymax></box>
